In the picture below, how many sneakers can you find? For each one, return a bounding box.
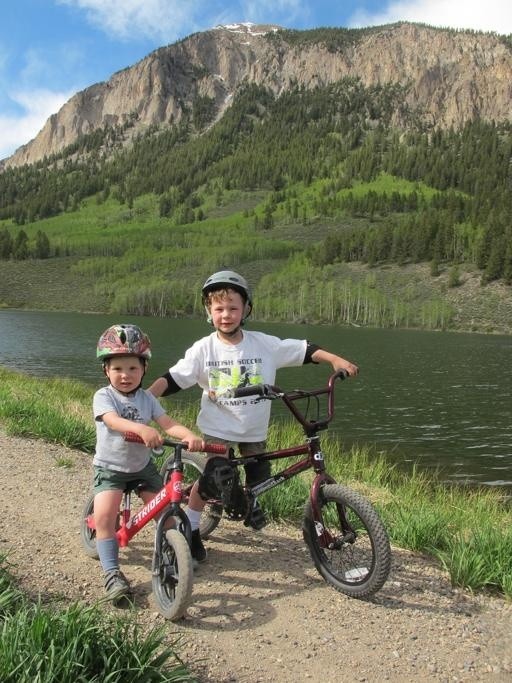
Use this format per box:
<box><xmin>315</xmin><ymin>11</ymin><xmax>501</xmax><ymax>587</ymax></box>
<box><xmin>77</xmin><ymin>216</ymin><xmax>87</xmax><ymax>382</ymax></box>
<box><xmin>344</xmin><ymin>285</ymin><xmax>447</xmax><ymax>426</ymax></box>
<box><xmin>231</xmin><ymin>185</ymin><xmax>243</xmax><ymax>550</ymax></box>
<box><xmin>162</xmin><ymin>529</ymin><xmax>208</xmax><ymax>570</ymax></box>
<box><xmin>103</xmin><ymin>569</ymin><xmax>130</xmax><ymax>606</ymax></box>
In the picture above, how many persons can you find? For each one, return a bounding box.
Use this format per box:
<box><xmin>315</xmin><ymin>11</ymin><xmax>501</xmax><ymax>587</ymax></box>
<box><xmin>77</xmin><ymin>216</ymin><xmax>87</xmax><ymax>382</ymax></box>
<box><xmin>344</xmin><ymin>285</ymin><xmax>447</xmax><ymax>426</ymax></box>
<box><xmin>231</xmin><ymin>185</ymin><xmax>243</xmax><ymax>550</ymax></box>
<box><xmin>92</xmin><ymin>323</ymin><xmax>206</xmax><ymax>606</ymax></box>
<box><xmin>145</xmin><ymin>270</ymin><xmax>360</xmax><ymax>564</ymax></box>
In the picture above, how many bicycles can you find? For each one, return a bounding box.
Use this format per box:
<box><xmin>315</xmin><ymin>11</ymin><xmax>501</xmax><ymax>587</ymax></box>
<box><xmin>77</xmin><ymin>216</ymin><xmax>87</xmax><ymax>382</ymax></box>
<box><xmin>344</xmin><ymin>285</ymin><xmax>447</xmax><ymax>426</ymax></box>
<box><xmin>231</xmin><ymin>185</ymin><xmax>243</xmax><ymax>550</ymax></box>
<box><xmin>158</xmin><ymin>366</ymin><xmax>394</xmax><ymax>598</ymax></box>
<box><xmin>77</xmin><ymin>429</ymin><xmax>229</xmax><ymax>624</ymax></box>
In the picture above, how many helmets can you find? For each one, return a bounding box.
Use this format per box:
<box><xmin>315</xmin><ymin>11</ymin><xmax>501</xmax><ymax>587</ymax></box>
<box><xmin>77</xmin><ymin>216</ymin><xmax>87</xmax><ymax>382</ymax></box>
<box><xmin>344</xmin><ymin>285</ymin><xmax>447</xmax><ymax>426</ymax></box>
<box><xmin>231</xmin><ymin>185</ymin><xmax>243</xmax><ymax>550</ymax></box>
<box><xmin>97</xmin><ymin>324</ymin><xmax>152</xmax><ymax>360</ymax></box>
<box><xmin>201</xmin><ymin>271</ymin><xmax>252</xmax><ymax>306</ymax></box>
